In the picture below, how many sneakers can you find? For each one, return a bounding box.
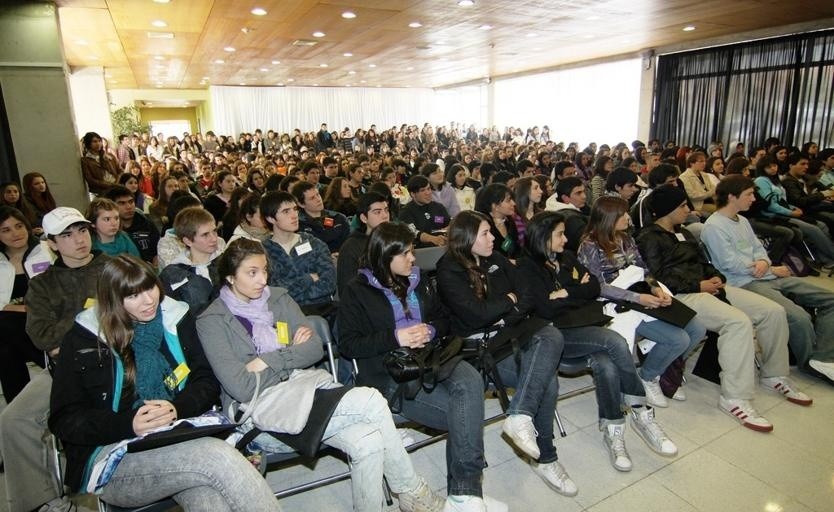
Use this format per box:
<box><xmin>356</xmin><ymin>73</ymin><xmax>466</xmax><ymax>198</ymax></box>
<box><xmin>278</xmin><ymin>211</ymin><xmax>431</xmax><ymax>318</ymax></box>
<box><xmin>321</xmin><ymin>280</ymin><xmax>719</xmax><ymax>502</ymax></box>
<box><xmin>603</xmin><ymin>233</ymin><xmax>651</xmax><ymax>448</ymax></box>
<box><xmin>601</xmin><ymin>423</ymin><xmax>633</xmax><ymax>471</ymax></box>
<box><xmin>399</xmin><ymin>476</ymin><xmax>447</xmax><ymax>511</ymax></box>
<box><xmin>637</xmin><ymin>367</ymin><xmax>669</xmax><ymax>408</ymax></box>
<box><xmin>660</xmin><ymin>358</ymin><xmax>686</xmax><ymax>400</ymax></box>
<box><xmin>758</xmin><ymin>376</ymin><xmax>813</xmax><ymax>405</ymax></box>
<box><xmin>444</xmin><ymin>495</ymin><xmax>487</xmax><ymax>511</ymax></box>
<box><xmin>717</xmin><ymin>394</ymin><xmax>773</xmax><ymax>432</ymax></box>
<box><xmin>628</xmin><ymin>405</ymin><xmax>678</xmax><ymax>456</ymax></box>
<box><xmin>482</xmin><ymin>492</ymin><xmax>508</xmax><ymax>511</ymax></box>
<box><xmin>531</xmin><ymin>458</ymin><xmax>578</xmax><ymax>497</ymax></box>
<box><xmin>502</xmin><ymin>414</ymin><xmax>540</xmax><ymax>459</ymax></box>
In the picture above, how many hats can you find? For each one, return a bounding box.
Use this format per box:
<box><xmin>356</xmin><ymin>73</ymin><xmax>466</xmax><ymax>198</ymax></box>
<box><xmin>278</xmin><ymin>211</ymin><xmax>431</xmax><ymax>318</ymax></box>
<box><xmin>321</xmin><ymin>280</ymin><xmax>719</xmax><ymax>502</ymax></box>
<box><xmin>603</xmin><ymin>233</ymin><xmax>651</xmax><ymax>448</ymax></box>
<box><xmin>41</xmin><ymin>206</ymin><xmax>92</xmax><ymax>238</ymax></box>
<box><xmin>707</xmin><ymin>144</ymin><xmax>723</xmax><ymax>158</ymax></box>
<box><xmin>643</xmin><ymin>184</ymin><xmax>687</xmax><ymax>222</ymax></box>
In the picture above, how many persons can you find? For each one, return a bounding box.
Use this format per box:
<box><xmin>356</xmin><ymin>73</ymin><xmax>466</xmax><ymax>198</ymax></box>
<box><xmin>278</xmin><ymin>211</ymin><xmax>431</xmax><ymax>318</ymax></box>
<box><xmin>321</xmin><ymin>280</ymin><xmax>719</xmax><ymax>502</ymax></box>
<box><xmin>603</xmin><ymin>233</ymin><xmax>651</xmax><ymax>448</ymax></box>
<box><xmin>0</xmin><ymin>124</ymin><xmax>834</xmax><ymax>510</ymax></box>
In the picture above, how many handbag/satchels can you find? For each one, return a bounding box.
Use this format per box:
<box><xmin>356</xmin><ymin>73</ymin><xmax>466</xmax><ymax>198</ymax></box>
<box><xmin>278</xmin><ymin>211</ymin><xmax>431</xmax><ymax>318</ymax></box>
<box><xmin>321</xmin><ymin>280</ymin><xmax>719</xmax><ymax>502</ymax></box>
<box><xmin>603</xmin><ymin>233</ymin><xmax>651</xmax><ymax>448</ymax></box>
<box><xmin>464</xmin><ymin>326</ymin><xmax>527</xmax><ymax>359</ymax></box>
<box><xmin>386</xmin><ymin>336</ymin><xmax>462</xmax><ymax>384</ymax></box>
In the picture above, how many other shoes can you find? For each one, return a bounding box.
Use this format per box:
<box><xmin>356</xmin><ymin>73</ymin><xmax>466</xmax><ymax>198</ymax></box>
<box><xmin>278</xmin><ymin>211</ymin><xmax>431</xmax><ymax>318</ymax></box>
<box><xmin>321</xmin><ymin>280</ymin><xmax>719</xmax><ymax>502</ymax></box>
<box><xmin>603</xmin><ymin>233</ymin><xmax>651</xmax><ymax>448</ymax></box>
<box><xmin>808</xmin><ymin>359</ymin><xmax>833</xmax><ymax>381</ymax></box>
<box><xmin>798</xmin><ymin>364</ymin><xmax>832</xmax><ymax>383</ymax></box>
